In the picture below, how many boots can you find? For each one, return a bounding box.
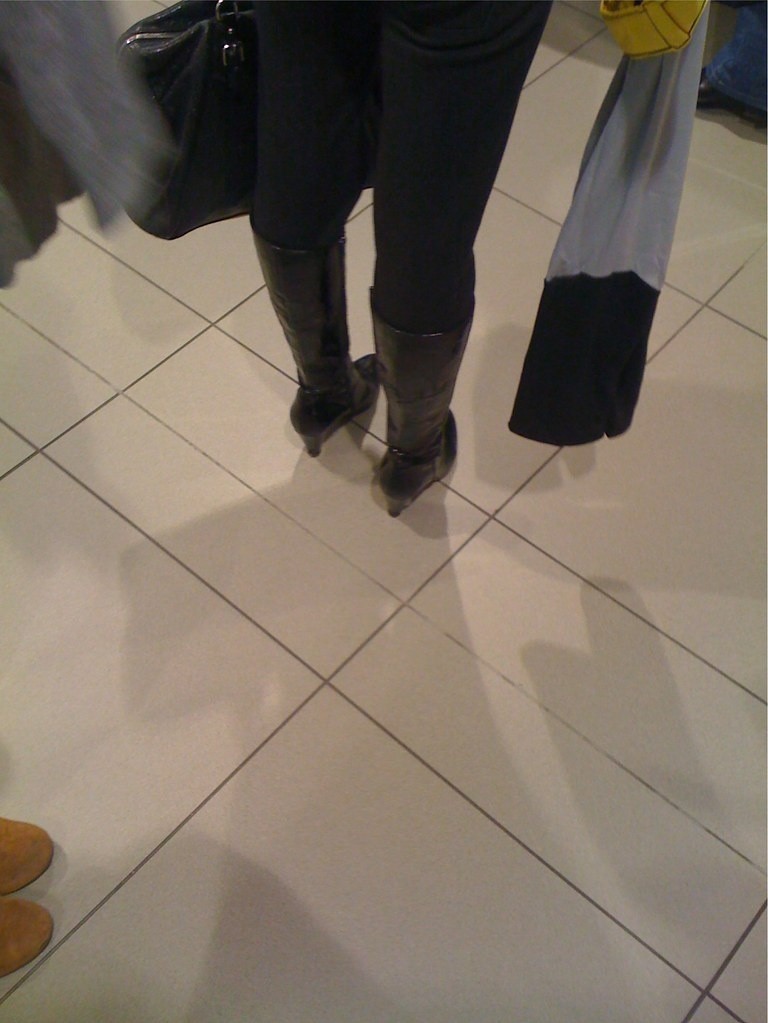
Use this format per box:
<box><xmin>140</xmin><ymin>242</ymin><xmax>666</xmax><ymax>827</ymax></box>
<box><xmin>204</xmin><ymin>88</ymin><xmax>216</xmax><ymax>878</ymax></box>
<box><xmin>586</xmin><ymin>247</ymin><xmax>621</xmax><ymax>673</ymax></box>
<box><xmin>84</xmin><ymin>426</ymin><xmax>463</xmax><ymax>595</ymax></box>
<box><xmin>370</xmin><ymin>286</ymin><xmax>474</xmax><ymax>519</ymax></box>
<box><xmin>250</xmin><ymin>214</ymin><xmax>381</xmax><ymax>461</ymax></box>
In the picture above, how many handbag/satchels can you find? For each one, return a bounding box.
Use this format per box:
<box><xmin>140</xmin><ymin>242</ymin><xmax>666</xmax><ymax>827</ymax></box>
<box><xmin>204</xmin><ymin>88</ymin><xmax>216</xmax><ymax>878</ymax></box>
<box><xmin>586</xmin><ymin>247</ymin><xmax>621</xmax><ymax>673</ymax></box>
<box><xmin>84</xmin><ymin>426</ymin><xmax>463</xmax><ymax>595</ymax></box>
<box><xmin>109</xmin><ymin>0</ymin><xmax>265</xmax><ymax>240</ymax></box>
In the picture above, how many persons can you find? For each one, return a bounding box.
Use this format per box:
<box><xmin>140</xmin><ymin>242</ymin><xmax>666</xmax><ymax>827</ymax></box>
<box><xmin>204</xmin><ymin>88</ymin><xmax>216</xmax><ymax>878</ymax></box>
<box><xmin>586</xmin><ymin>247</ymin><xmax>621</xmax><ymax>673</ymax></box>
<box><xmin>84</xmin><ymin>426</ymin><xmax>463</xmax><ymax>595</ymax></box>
<box><xmin>696</xmin><ymin>0</ymin><xmax>767</xmax><ymax>129</ymax></box>
<box><xmin>219</xmin><ymin>0</ymin><xmax>556</xmax><ymax>518</ymax></box>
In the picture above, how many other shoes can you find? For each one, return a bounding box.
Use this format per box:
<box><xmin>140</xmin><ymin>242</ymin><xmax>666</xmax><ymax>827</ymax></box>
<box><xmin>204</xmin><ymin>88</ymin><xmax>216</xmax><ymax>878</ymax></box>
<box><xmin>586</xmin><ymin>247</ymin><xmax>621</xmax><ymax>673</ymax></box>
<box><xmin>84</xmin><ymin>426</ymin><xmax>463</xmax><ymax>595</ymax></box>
<box><xmin>0</xmin><ymin>815</ymin><xmax>55</xmax><ymax>897</ymax></box>
<box><xmin>693</xmin><ymin>67</ymin><xmax>767</xmax><ymax>128</ymax></box>
<box><xmin>0</xmin><ymin>897</ymin><xmax>53</xmax><ymax>980</ymax></box>
